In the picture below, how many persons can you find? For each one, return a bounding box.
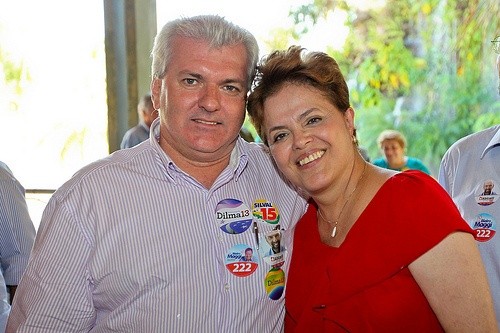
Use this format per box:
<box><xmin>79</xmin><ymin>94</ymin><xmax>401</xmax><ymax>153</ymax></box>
<box><xmin>478</xmin><ymin>180</ymin><xmax>498</xmax><ymax>195</ymax></box>
<box><xmin>264</xmin><ymin>232</ymin><xmax>288</xmax><ymax>256</ymax></box>
<box><xmin>238</xmin><ymin>248</ymin><xmax>258</xmax><ymax>262</ymax></box>
<box><xmin>0</xmin><ymin>14</ymin><xmax>500</xmax><ymax>333</ymax></box>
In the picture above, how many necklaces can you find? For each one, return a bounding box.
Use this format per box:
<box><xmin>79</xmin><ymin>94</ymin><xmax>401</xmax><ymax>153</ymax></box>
<box><xmin>318</xmin><ymin>161</ymin><xmax>367</xmax><ymax>238</ymax></box>
